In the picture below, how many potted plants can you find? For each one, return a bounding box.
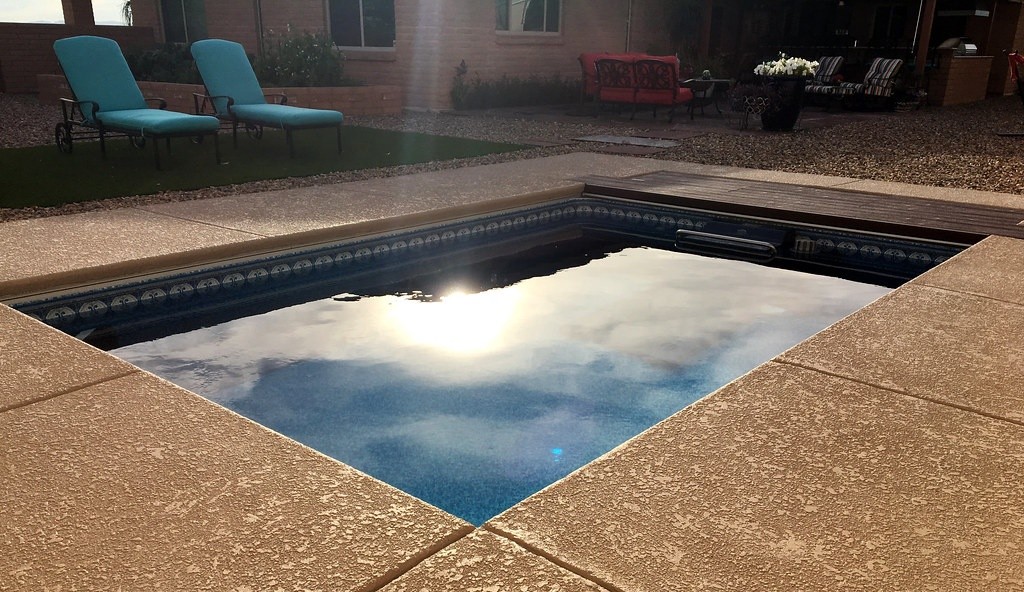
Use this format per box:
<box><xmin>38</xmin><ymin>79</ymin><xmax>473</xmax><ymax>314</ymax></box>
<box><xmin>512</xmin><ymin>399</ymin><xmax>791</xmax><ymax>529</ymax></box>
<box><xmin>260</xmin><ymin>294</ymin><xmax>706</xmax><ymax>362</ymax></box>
<box><xmin>739</xmin><ymin>86</ymin><xmax>769</xmax><ymax>129</ymax></box>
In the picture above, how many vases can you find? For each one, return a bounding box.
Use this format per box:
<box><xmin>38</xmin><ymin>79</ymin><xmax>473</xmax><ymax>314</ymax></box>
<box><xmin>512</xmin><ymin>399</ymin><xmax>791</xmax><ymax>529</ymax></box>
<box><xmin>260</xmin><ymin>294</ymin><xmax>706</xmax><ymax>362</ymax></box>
<box><xmin>833</xmin><ymin>79</ymin><xmax>840</xmax><ymax>86</ymax></box>
<box><xmin>754</xmin><ymin>78</ymin><xmax>807</xmax><ymax>132</ymax></box>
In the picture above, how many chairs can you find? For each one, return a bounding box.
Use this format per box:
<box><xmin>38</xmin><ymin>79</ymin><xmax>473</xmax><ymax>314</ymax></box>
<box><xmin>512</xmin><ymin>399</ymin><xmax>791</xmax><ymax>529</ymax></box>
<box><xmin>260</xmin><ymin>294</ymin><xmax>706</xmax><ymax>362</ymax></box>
<box><xmin>187</xmin><ymin>39</ymin><xmax>343</xmax><ymax>160</ymax></box>
<box><xmin>52</xmin><ymin>34</ymin><xmax>221</xmax><ymax>172</ymax></box>
<box><xmin>838</xmin><ymin>57</ymin><xmax>904</xmax><ymax>110</ymax></box>
<box><xmin>804</xmin><ymin>55</ymin><xmax>843</xmax><ymax>85</ymax></box>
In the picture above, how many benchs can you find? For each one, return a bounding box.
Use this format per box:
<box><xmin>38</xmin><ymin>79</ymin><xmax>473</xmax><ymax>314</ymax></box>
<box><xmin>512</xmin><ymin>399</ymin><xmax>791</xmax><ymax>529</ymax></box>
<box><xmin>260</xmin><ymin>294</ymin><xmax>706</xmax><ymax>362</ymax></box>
<box><xmin>805</xmin><ymin>85</ymin><xmax>855</xmax><ymax>112</ymax></box>
<box><xmin>577</xmin><ymin>52</ymin><xmax>696</xmax><ymax>125</ymax></box>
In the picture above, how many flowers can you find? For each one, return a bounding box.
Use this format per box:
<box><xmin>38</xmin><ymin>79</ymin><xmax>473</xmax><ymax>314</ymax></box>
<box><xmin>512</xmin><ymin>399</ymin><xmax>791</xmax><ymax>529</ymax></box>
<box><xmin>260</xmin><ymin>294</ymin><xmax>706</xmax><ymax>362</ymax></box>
<box><xmin>750</xmin><ymin>55</ymin><xmax>820</xmax><ymax>78</ymax></box>
<box><xmin>829</xmin><ymin>73</ymin><xmax>845</xmax><ymax>82</ymax></box>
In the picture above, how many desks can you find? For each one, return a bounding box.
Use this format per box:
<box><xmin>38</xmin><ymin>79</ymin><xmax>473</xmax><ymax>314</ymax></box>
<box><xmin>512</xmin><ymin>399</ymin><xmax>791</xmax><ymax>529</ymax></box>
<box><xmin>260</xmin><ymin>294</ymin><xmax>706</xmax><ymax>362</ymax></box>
<box><xmin>687</xmin><ymin>79</ymin><xmax>732</xmax><ymax>114</ymax></box>
